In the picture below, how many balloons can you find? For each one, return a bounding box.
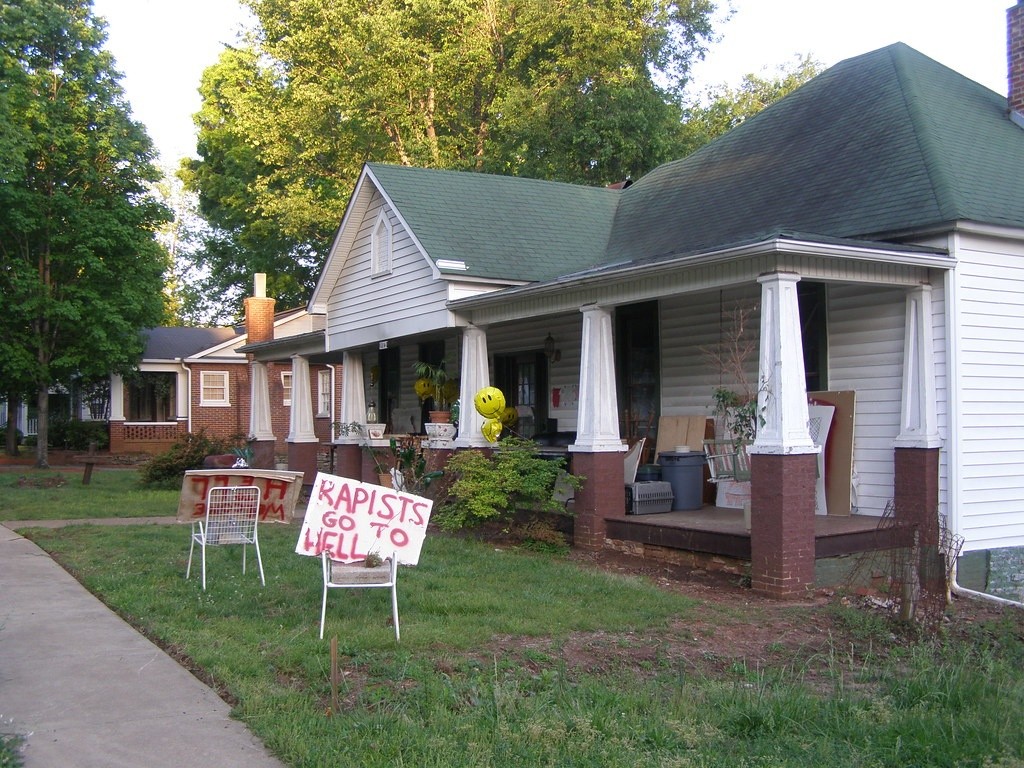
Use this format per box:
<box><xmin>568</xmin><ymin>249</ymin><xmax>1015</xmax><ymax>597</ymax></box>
<box><xmin>474</xmin><ymin>387</ymin><xmax>506</xmax><ymax>419</ymax></box>
<box><xmin>415</xmin><ymin>378</ymin><xmax>459</xmax><ymax>403</ymax></box>
<box><xmin>481</xmin><ymin>419</ymin><xmax>502</xmax><ymax>443</ymax></box>
<box><xmin>502</xmin><ymin>407</ymin><xmax>518</xmax><ymax>427</ymax></box>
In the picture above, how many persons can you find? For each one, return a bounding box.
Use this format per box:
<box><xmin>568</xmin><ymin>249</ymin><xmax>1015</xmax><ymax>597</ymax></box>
<box><xmin>422</xmin><ymin>398</ymin><xmax>433</xmax><ymax>433</ymax></box>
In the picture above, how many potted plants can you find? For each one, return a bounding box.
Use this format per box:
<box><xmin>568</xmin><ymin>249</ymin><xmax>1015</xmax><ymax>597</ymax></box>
<box><xmin>328</xmin><ymin>421</ymin><xmax>392</xmax><ymax>488</ymax></box>
<box><xmin>410</xmin><ymin>351</ymin><xmax>454</xmax><ymax>422</ymax></box>
<box><xmin>706</xmin><ymin>385</ymin><xmax>769</xmax><ymax>533</ymax></box>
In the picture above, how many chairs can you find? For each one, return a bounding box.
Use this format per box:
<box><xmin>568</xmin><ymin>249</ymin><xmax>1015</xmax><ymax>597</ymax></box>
<box><xmin>319</xmin><ymin>550</ymin><xmax>400</xmax><ymax>641</ymax></box>
<box><xmin>186</xmin><ymin>486</ymin><xmax>265</xmax><ymax>591</ymax></box>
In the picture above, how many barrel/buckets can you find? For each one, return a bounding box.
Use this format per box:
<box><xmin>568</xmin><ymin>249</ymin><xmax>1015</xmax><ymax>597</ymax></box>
<box><xmin>635</xmin><ymin>450</ymin><xmax>707</xmax><ymax>512</ymax></box>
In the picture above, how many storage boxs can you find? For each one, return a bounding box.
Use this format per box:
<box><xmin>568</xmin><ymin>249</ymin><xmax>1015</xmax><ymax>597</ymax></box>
<box><xmin>624</xmin><ymin>481</ymin><xmax>674</xmax><ymax>515</ymax></box>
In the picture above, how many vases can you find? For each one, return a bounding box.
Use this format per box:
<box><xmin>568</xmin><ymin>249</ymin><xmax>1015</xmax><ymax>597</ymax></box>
<box><xmin>366</xmin><ymin>424</ymin><xmax>386</xmax><ymax>439</ymax></box>
<box><xmin>425</xmin><ymin>423</ymin><xmax>457</xmax><ymax>443</ymax></box>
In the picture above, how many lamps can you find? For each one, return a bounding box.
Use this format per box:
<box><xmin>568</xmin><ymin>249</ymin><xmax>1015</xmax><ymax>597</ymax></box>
<box><xmin>544</xmin><ymin>331</ymin><xmax>561</xmax><ymax>364</ymax></box>
<box><xmin>366</xmin><ymin>366</ymin><xmax>380</xmax><ymax>422</ymax></box>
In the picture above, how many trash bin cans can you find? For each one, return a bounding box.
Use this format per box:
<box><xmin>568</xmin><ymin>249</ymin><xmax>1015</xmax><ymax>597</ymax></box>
<box><xmin>637</xmin><ymin>449</ymin><xmax>707</xmax><ymax>510</ymax></box>
